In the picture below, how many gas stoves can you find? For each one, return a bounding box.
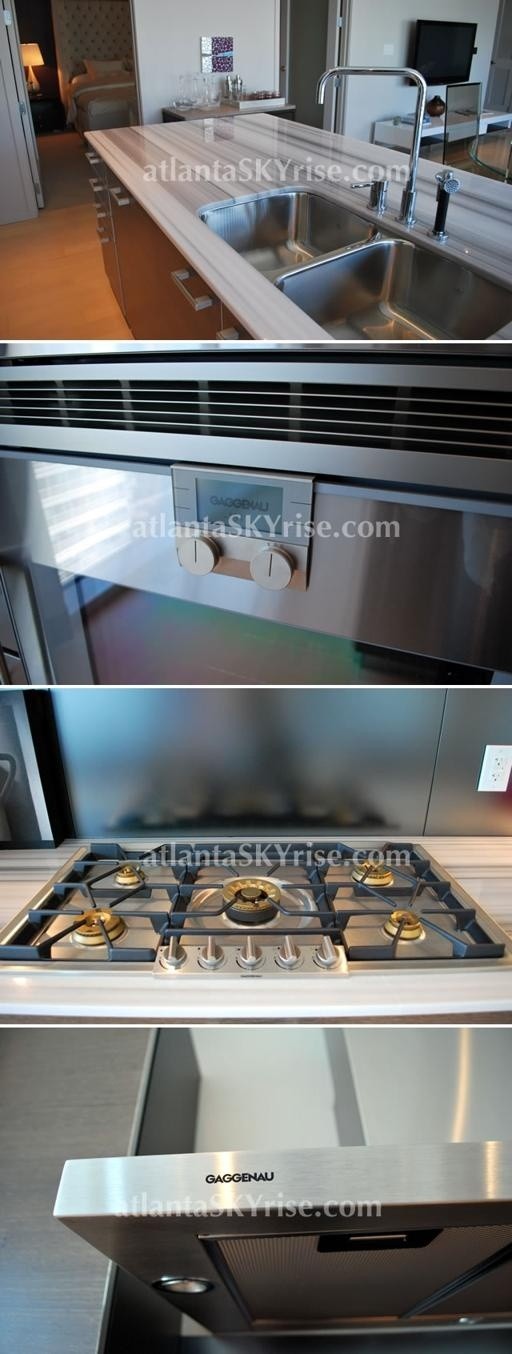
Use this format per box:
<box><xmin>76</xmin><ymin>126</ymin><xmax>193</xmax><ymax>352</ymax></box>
<box><xmin>0</xmin><ymin>844</ymin><xmax>512</xmax><ymax>974</ymax></box>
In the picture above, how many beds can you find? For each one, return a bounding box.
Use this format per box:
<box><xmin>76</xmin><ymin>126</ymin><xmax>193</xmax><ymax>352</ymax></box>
<box><xmin>65</xmin><ymin>74</ymin><xmax>138</xmax><ymax>146</ymax></box>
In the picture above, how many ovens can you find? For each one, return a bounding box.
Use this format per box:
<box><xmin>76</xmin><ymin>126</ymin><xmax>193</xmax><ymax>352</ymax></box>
<box><xmin>1</xmin><ymin>342</ymin><xmax>512</xmax><ymax>684</ymax></box>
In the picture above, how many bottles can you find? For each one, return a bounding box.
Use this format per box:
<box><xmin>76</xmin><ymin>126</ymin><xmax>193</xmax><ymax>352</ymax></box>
<box><xmin>427</xmin><ymin>95</ymin><xmax>445</xmax><ymax>118</ymax></box>
<box><xmin>224</xmin><ymin>73</ymin><xmax>244</xmax><ymax>100</ymax></box>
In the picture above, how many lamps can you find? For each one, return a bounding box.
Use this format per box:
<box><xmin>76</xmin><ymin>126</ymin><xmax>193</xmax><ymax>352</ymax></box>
<box><xmin>20</xmin><ymin>43</ymin><xmax>46</xmax><ymax>94</ymax></box>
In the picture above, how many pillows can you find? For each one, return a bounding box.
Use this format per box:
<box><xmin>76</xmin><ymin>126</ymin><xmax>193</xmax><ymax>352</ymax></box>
<box><xmin>84</xmin><ymin>58</ymin><xmax>123</xmax><ymax>80</ymax></box>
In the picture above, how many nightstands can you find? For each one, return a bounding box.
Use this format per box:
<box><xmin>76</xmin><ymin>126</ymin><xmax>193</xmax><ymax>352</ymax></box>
<box><xmin>29</xmin><ymin>86</ymin><xmax>67</xmax><ymax>133</ymax></box>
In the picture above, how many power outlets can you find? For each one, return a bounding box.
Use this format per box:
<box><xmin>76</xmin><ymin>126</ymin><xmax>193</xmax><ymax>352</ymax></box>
<box><xmin>476</xmin><ymin>744</ymin><xmax>512</xmax><ymax>793</ymax></box>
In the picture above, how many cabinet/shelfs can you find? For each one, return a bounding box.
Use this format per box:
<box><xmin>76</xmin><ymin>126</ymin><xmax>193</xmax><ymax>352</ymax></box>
<box><xmin>84</xmin><ymin>143</ymin><xmax>254</xmax><ymax>339</ymax></box>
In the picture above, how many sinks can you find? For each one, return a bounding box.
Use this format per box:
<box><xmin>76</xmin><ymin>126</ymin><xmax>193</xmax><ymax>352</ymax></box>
<box><xmin>273</xmin><ymin>239</ymin><xmax>512</xmax><ymax>339</ymax></box>
<box><xmin>199</xmin><ymin>190</ymin><xmax>374</xmax><ymax>271</ymax></box>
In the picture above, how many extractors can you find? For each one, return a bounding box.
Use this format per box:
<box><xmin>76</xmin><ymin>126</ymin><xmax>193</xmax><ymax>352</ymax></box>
<box><xmin>52</xmin><ymin>1026</ymin><xmax>510</xmax><ymax>1337</ymax></box>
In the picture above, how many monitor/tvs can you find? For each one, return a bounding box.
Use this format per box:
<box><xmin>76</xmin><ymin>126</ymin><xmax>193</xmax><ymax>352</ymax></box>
<box><xmin>409</xmin><ymin>19</ymin><xmax>478</xmax><ymax>86</ymax></box>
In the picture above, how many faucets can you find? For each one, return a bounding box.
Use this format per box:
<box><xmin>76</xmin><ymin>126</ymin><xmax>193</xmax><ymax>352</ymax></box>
<box><xmin>314</xmin><ymin>66</ymin><xmax>429</xmax><ymax>188</ymax></box>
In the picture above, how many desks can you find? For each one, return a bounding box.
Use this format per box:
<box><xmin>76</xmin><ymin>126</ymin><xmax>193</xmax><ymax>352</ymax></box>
<box><xmin>161</xmin><ymin>103</ymin><xmax>297</xmax><ymax>122</ymax></box>
<box><xmin>372</xmin><ymin>107</ymin><xmax>512</xmax><ymax>155</ymax></box>
